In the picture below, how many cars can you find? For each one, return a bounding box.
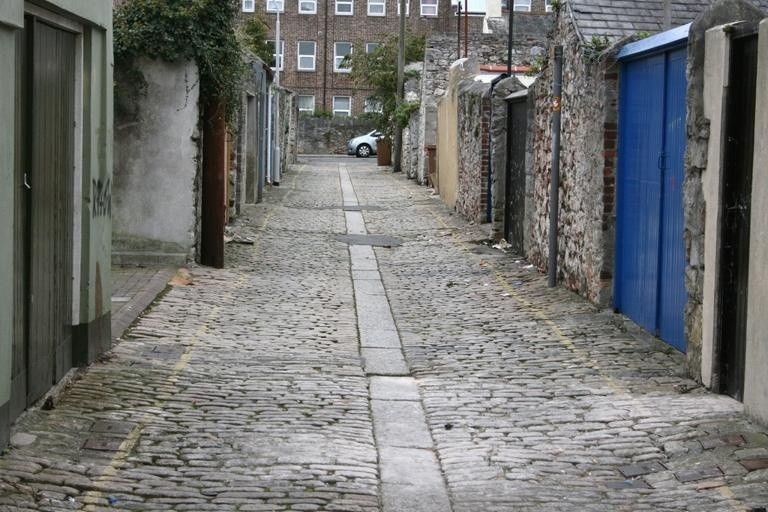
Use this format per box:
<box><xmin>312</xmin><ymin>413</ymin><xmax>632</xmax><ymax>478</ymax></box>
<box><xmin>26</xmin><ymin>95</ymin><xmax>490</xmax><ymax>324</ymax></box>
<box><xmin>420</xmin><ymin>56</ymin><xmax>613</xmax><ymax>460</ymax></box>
<box><xmin>346</xmin><ymin>129</ymin><xmax>385</xmax><ymax>158</ymax></box>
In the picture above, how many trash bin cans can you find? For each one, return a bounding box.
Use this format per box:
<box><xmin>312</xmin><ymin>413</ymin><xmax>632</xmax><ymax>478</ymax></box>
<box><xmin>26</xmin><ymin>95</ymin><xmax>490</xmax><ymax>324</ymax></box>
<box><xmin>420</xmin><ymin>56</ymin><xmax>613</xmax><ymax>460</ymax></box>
<box><xmin>376</xmin><ymin>139</ymin><xmax>392</xmax><ymax>166</ymax></box>
<box><xmin>427</xmin><ymin>145</ymin><xmax>437</xmax><ymax>187</ymax></box>
